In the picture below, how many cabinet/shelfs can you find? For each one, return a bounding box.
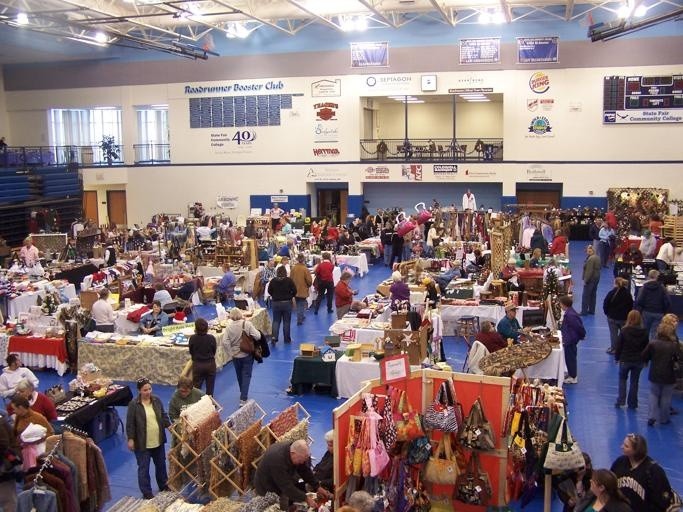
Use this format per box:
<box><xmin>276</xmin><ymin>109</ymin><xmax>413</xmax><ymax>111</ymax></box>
<box><xmin>334</xmin><ymin>369</ymin><xmax>540</xmax><ymax>512</ymax></box>
<box><xmin>208</xmin><ymin>400</ymin><xmax>267</xmax><ymax>498</ymax></box>
<box><xmin>251</xmin><ymin>402</ymin><xmax>311</xmax><ymax>469</ymax></box>
<box><xmin>166</xmin><ymin>395</ymin><xmax>223</xmax><ymax>501</ymax></box>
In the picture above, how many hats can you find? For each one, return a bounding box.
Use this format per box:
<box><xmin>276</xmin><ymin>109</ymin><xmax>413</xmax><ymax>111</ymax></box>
<box><xmin>504</xmin><ymin>302</ymin><xmax>518</xmax><ymax>309</ymax></box>
<box><xmin>452</xmin><ymin>260</ymin><xmax>462</xmax><ymax>265</ymax></box>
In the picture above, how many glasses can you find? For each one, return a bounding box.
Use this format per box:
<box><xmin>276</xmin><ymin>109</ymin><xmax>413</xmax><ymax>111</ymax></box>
<box><xmin>138</xmin><ymin>378</ymin><xmax>149</xmax><ymax>384</ymax></box>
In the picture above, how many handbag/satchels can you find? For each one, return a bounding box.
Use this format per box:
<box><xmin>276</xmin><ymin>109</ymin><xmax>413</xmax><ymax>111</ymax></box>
<box><xmin>170</xmin><ymin>245</ymin><xmax>180</xmax><ymax>259</ymax></box>
<box><xmin>85</xmin><ymin>409</ymin><xmax>119</xmax><ymax>443</ymax></box>
<box><xmin>345</xmin><ymin>378</ymin><xmax>494</xmax><ymax>511</ymax></box>
<box><xmin>45</xmin><ymin>383</ymin><xmax>66</xmax><ymax>403</ymax></box>
<box><xmin>501</xmin><ymin>380</ymin><xmax>585</xmax><ymax>510</ymax></box>
<box><xmin>240</xmin><ymin>330</ymin><xmax>255</xmax><ymax>353</ymax></box>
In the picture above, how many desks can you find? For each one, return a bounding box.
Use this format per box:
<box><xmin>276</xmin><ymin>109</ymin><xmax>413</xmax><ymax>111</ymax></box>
<box><xmin>566</xmin><ymin>214</ymin><xmax>683</xmax><ymax>317</ymax></box>
<box><xmin>1</xmin><ymin>214</ymin><xmax>384</xmax><ymax>387</ymax></box>
<box><xmin>52</xmin><ymin>384</ymin><xmax>133</xmax><ymax>443</ymax></box>
<box><xmin>290</xmin><ymin>212</ymin><xmax>565</xmax><ymax>400</ymax></box>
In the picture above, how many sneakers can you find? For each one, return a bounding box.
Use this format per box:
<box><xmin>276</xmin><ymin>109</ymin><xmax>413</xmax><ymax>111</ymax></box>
<box><xmin>565</xmin><ymin>376</ymin><xmax>576</xmax><ymax>384</ymax></box>
